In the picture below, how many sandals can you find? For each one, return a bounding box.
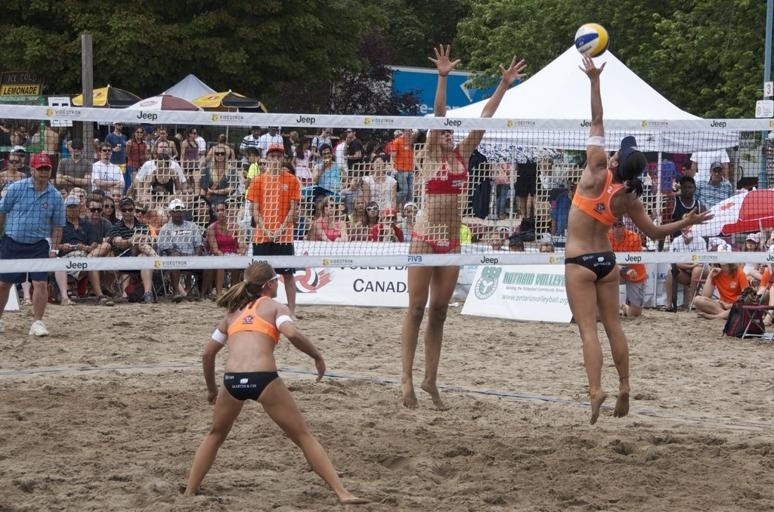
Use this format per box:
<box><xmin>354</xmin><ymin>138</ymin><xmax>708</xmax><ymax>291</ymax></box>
<box><xmin>68</xmin><ymin>290</ymin><xmax>78</xmax><ymax>302</ymax></box>
<box><xmin>98</xmin><ymin>295</ymin><xmax>114</xmax><ymax>307</ymax></box>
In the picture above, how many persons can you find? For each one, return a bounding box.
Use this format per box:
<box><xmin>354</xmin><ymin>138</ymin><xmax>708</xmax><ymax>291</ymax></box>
<box><xmin>398</xmin><ymin>42</ymin><xmax>530</xmax><ymax>412</ymax></box>
<box><xmin>560</xmin><ymin>49</ymin><xmax>716</xmax><ymax>427</ymax></box>
<box><xmin>0</xmin><ymin>114</ymin><xmax>774</xmax><ymax>323</ymax></box>
<box><xmin>0</xmin><ymin>152</ymin><xmax>65</xmax><ymax>339</ymax></box>
<box><xmin>181</xmin><ymin>258</ymin><xmax>373</xmax><ymax>506</ymax></box>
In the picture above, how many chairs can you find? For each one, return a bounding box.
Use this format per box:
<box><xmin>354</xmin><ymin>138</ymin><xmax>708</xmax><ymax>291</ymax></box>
<box><xmin>740</xmin><ymin>272</ymin><xmax>774</xmax><ymax>341</ymax></box>
<box><xmin>688</xmin><ymin>264</ymin><xmax>719</xmax><ymax>311</ymax></box>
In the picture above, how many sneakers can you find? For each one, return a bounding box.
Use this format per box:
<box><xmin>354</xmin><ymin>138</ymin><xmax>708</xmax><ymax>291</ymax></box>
<box><xmin>29</xmin><ymin>319</ymin><xmax>50</xmax><ymax>337</ymax></box>
<box><xmin>116</xmin><ymin>297</ymin><xmax>133</xmax><ymax>304</ymax></box>
<box><xmin>145</xmin><ymin>292</ymin><xmax>155</xmax><ymax>304</ymax></box>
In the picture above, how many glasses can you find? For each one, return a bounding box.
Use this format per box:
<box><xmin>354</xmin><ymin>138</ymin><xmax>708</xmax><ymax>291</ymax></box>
<box><xmin>262</xmin><ymin>274</ymin><xmax>281</xmax><ymax>289</ymax></box>
<box><xmin>0</xmin><ymin>120</ymin><xmax>420</xmax><ymax>227</ymax></box>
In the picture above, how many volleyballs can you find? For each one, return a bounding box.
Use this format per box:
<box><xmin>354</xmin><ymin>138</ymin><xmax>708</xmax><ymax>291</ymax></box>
<box><xmin>575</xmin><ymin>23</ymin><xmax>609</xmax><ymax>57</ymax></box>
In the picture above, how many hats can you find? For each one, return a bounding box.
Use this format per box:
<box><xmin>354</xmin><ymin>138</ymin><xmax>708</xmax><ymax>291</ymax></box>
<box><xmin>746</xmin><ymin>234</ymin><xmax>760</xmax><ymax>242</ymax></box>
<box><xmin>619</xmin><ymin>136</ymin><xmax>640</xmax><ymax>178</ymax></box>
<box><xmin>710</xmin><ymin>163</ymin><xmax>722</xmax><ymax>169</ymax></box>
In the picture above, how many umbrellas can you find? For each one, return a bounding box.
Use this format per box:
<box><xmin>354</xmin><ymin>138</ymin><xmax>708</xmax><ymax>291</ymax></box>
<box><xmin>72</xmin><ymin>86</ymin><xmax>143</xmax><ymax>136</ymax></box>
<box><xmin>122</xmin><ymin>93</ymin><xmax>206</xmax><ymax>130</ymax></box>
<box><xmin>189</xmin><ymin>89</ymin><xmax>269</xmax><ymax>144</ymax></box>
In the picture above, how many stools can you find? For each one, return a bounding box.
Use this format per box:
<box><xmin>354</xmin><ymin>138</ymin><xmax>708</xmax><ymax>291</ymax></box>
<box><xmin>113</xmin><ymin>270</ymin><xmax>201</xmax><ymax>301</ymax></box>
